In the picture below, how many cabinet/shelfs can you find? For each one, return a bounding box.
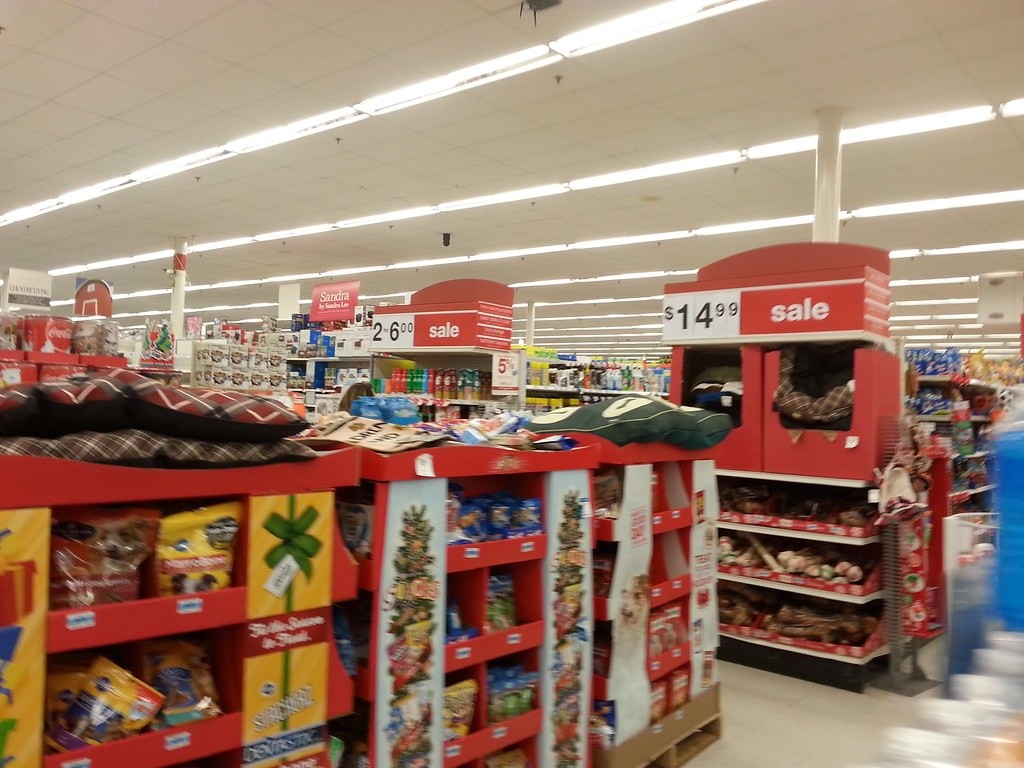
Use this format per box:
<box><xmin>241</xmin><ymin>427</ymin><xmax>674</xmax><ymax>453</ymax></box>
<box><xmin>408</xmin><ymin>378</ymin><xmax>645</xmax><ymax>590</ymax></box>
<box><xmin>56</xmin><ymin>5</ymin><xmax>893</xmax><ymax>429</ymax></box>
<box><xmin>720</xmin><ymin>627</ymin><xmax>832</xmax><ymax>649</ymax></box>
<box><xmin>520</xmin><ymin>350</ymin><xmax>668</xmax><ymax>410</ymax></box>
<box><xmin>536</xmin><ymin>427</ymin><xmax>723</xmax><ymax>768</ymax></box>
<box><xmin>668</xmin><ymin>328</ymin><xmax>907</xmax><ymax>693</ymax></box>
<box><xmin>285</xmin><ymin>358</ymin><xmax>371</xmax><ymax>407</ymax></box>
<box><xmin>0</xmin><ymin>437</ymin><xmax>363</xmax><ymax>768</ymax></box>
<box><xmin>294</xmin><ymin>439</ymin><xmax>600</xmax><ymax>768</ymax></box>
<box><xmin>369</xmin><ymin>348</ymin><xmax>521</xmax><ymax>413</ymax></box>
<box><xmin>903</xmin><ymin>375</ymin><xmax>998</xmax><ymax>500</ymax></box>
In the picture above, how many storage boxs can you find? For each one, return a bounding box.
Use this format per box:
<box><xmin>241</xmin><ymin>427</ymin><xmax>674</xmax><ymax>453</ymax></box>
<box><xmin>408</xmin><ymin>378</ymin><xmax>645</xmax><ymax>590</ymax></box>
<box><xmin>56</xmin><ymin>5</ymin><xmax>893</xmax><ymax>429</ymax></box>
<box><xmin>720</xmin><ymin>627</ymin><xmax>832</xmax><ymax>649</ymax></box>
<box><xmin>0</xmin><ymin>315</ymin><xmax>119</xmax><ymax>387</ymax></box>
<box><xmin>669</xmin><ymin>345</ymin><xmax>765</xmax><ymax>472</ymax></box>
<box><xmin>763</xmin><ymin>348</ymin><xmax>900</xmax><ymax>479</ymax></box>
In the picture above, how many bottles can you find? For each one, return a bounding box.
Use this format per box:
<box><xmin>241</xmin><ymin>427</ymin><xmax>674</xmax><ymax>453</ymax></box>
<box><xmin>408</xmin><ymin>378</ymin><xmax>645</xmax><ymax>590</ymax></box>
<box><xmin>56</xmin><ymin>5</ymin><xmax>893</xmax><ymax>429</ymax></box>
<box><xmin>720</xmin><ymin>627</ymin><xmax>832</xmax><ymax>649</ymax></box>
<box><xmin>527</xmin><ymin>349</ymin><xmax>671</xmax><ymax>393</ymax></box>
